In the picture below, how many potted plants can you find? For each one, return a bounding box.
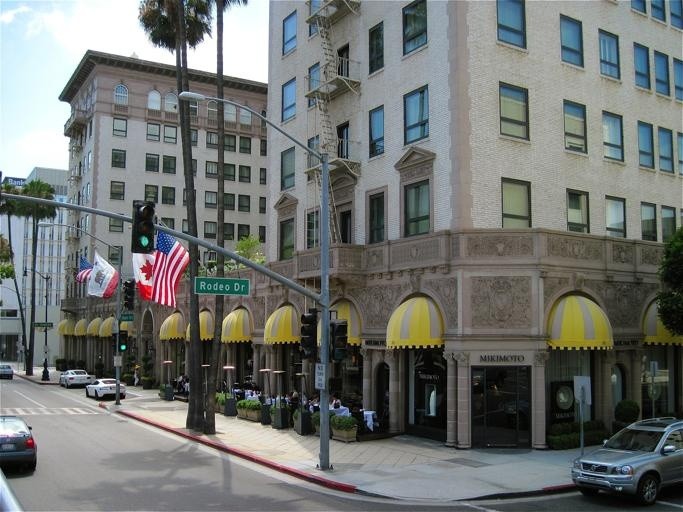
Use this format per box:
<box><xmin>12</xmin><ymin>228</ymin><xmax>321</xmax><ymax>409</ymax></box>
<box><xmin>215</xmin><ymin>392</ymin><xmax>358</xmax><ymax>444</ymax></box>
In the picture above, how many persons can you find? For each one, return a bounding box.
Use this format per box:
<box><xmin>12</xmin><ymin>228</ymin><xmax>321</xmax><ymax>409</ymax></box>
<box><xmin>172</xmin><ymin>374</ymin><xmax>365</xmax><ymax>435</ymax></box>
<box><xmin>132</xmin><ymin>364</ymin><xmax>140</xmax><ymax>386</ymax></box>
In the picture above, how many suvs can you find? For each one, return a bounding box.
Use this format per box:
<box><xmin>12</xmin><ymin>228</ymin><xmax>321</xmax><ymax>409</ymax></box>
<box><xmin>572</xmin><ymin>416</ymin><xmax>682</xmax><ymax>504</ymax></box>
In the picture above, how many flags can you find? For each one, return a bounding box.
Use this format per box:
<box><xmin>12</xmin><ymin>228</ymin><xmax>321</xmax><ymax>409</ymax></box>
<box><xmin>85</xmin><ymin>248</ymin><xmax>120</xmax><ymax>298</ymax></box>
<box><xmin>131</xmin><ymin>250</ymin><xmax>158</xmax><ymax>301</ymax></box>
<box><xmin>149</xmin><ymin>224</ymin><xmax>189</xmax><ymax>309</ymax></box>
<box><xmin>74</xmin><ymin>255</ymin><xmax>93</xmax><ymax>283</ymax></box>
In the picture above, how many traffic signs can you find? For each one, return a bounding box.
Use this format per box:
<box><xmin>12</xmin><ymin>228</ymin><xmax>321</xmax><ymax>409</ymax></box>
<box><xmin>195</xmin><ymin>276</ymin><xmax>250</xmax><ymax>297</ymax></box>
<box><xmin>119</xmin><ymin>313</ymin><xmax>134</xmax><ymax>322</ymax></box>
<box><xmin>30</xmin><ymin>321</ymin><xmax>53</xmax><ymax>328</ymax></box>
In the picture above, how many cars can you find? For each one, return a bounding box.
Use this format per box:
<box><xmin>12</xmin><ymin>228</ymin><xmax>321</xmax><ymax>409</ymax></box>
<box><xmin>0</xmin><ymin>365</ymin><xmax>13</xmax><ymax>380</ymax></box>
<box><xmin>85</xmin><ymin>378</ymin><xmax>126</xmax><ymax>400</ymax></box>
<box><xmin>58</xmin><ymin>369</ymin><xmax>92</xmax><ymax>388</ymax></box>
<box><xmin>0</xmin><ymin>416</ymin><xmax>38</xmax><ymax>476</ymax></box>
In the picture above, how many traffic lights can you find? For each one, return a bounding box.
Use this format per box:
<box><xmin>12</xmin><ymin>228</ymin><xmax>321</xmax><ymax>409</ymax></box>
<box><xmin>119</xmin><ymin>330</ymin><xmax>128</xmax><ymax>352</ymax></box>
<box><xmin>124</xmin><ymin>277</ymin><xmax>135</xmax><ymax>311</ymax></box>
<box><xmin>131</xmin><ymin>199</ymin><xmax>155</xmax><ymax>254</ymax></box>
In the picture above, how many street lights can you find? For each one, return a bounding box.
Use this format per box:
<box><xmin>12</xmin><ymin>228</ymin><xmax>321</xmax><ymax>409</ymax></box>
<box><xmin>22</xmin><ymin>267</ymin><xmax>51</xmax><ymax>382</ymax></box>
<box><xmin>179</xmin><ymin>88</ymin><xmax>337</xmax><ymax>473</ymax></box>
<box><xmin>37</xmin><ymin>221</ymin><xmax>124</xmax><ymax>406</ymax></box>
